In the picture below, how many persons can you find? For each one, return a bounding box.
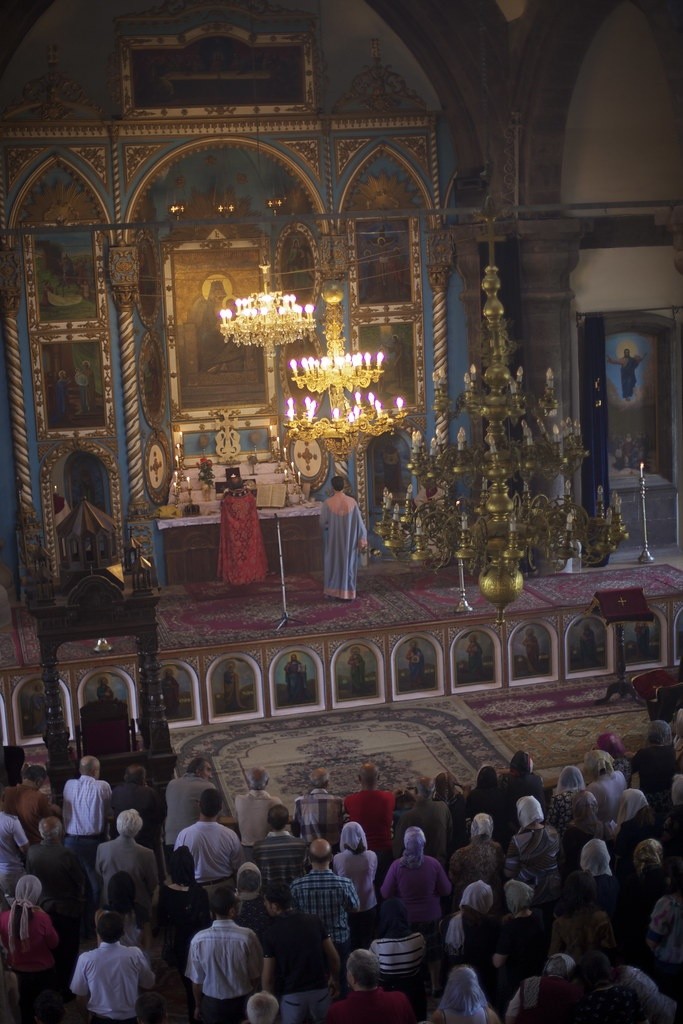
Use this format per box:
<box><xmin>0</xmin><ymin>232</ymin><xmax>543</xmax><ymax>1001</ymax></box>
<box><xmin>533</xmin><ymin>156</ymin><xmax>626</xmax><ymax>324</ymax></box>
<box><xmin>319</xmin><ymin>476</ymin><xmax>369</xmax><ymax>604</ymax></box>
<box><xmin>1</xmin><ymin>712</ymin><xmax>683</xmax><ymax>1022</ymax></box>
<box><xmin>219</xmin><ymin>473</ymin><xmax>264</xmax><ymax>584</ymax></box>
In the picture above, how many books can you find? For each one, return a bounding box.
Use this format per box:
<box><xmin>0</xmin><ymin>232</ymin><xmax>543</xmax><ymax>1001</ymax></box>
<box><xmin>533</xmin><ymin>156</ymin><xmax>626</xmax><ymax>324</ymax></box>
<box><xmin>255</xmin><ymin>483</ymin><xmax>287</xmax><ymax>509</ymax></box>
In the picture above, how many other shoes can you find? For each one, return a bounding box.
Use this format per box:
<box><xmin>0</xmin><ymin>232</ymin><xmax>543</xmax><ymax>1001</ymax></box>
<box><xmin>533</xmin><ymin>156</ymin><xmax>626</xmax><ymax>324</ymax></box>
<box><xmin>61</xmin><ymin>991</ymin><xmax>77</xmax><ymax>1003</ymax></box>
<box><xmin>432</xmin><ymin>985</ymin><xmax>445</xmax><ymax>999</ymax></box>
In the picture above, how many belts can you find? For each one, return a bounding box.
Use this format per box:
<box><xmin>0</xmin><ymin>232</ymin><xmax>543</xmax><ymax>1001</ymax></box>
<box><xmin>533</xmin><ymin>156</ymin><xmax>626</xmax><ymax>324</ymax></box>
<box><xmin>65</xmin><ymin>832</ymin><xmax>99</xmax><ymax>840</ymax></box>
<box><xmin>197</xmin><ymin>873</ymin><xmax>234</xmax><ymax>887</ymax></box>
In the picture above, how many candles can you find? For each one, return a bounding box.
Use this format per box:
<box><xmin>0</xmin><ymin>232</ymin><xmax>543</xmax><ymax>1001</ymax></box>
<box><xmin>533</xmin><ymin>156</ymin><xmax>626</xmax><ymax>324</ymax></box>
<box><xmin>284</xmin><ymin>448</ymin><xmax>286</xmax><ymax>461</ymax></box>
<box><xmin>173</xmin><ymin>483</ymin><xmax>176</xmax><ymax>496</ymax></box>
<box><xmin>187</xmin><ymin>477</ymin><xmax>190</xmax><ymax>489</ymax></box>
<box><xmin>175</xmin><ymin>455</ymin><xmax>179</xmax><ymax>468</ymax></box>
<box><xmin>298</xmin><ymin>472</ymin><xmax>301</xmax><ymax>485</ymax></box>
<box><xmin>284</xmin><ymin>469</ymin><xmax>288</xmax><ymax>481</ymax></box>
<box><xmin>175</xmin><ymin>472</ymin><xmax>177</xmax><ymax>482</ymax></box>
<box><xmin>277</xmin><ymin>437</ymin><xmax>279</xmax><ymax>441</ymax></box>
<box><xmin>639</xmin><ymin>462</ymin><xmax>644</xmax><ymax>476</ymax></box>
<box><xmin>291</xmin><ymin>462</ymin><xmax>294</xmax><ymax>474</ymax></box>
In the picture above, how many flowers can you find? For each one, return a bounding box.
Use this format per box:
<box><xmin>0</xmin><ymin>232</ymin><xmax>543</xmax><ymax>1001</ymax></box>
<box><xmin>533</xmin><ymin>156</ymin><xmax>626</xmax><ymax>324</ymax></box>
<box><xmin>195</xmin><ymin>457</ymin><xmax>215</xmax><ymax>485</ymax></box>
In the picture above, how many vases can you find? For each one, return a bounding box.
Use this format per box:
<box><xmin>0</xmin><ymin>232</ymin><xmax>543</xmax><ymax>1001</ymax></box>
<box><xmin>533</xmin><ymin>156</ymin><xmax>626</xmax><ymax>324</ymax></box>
<box><xmin>203</xmin><ymin>483</ymin><xmax>210</xmax><ymax>501</ymax></box>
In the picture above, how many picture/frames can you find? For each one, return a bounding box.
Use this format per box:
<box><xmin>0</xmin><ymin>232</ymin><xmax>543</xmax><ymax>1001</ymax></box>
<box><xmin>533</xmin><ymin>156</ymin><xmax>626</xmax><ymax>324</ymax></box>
<box><xmin>21</xmin><ymin>220</ymin><xmax>110</xmax><ymax>332</ymax></box>
<box><xmin>29</xmin><ymin>334</ymin><xmax>116</xmax><ymax>441</ymax></box>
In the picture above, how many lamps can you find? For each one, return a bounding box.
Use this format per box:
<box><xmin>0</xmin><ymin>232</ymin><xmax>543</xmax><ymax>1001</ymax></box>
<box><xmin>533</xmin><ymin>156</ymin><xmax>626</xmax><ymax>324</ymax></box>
<box><xmin>370</xmin><ymin>0</ymin><xmax>629</xmax><ymax>628</ymax></box>
<box><xmin>282</xmin><ymin>0</ymin><xmax>408</xmax><ymax>462</ymax></box>
<box><xmin>218</xmin><ymin>0</ymin><xmax>317</xmax><ymax>347</ymax></box>
<box><xmin>290</xmin><ymin>0</ymin><xmax>385</xmax><ymax>393</ymax></box>
